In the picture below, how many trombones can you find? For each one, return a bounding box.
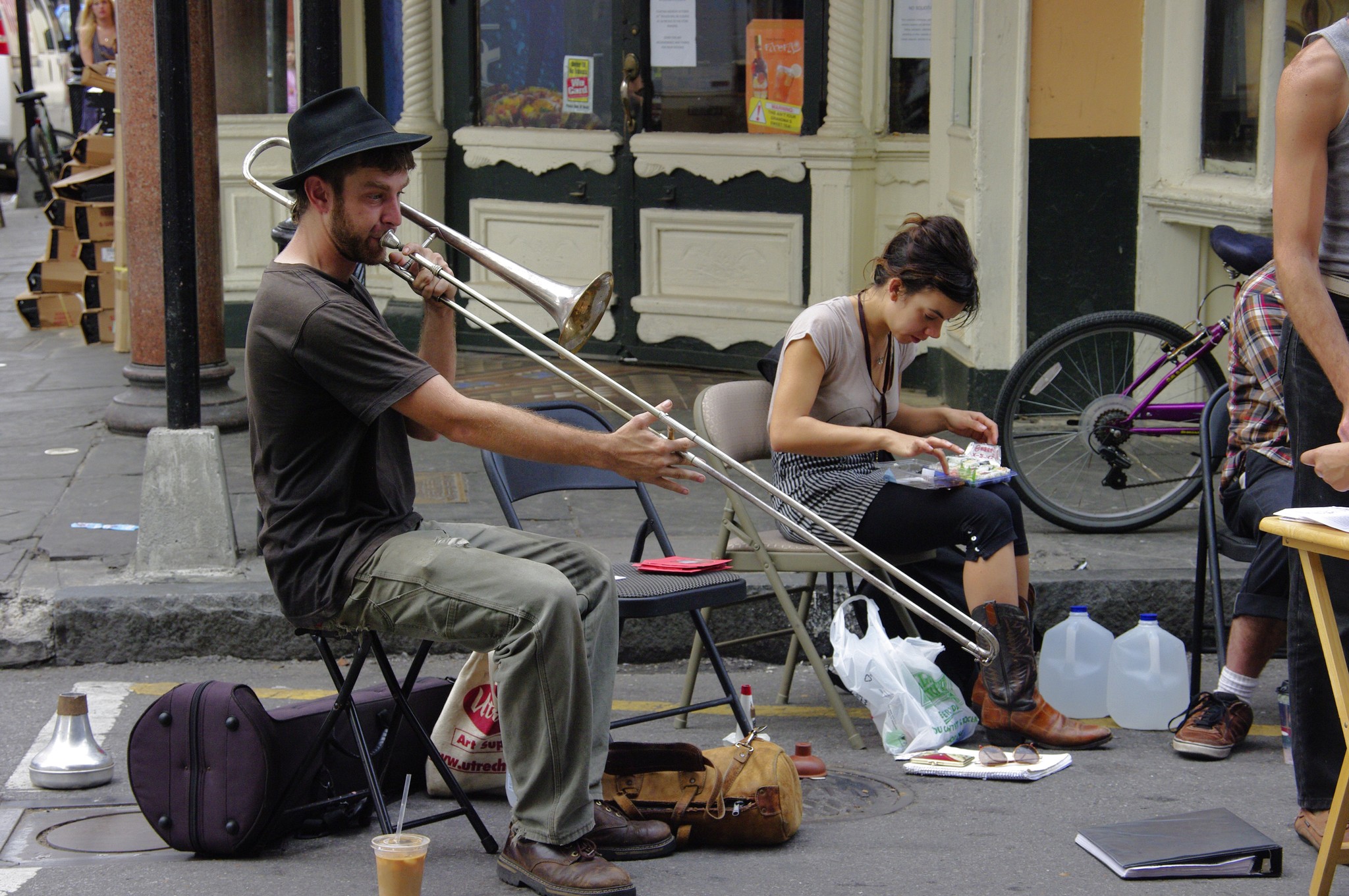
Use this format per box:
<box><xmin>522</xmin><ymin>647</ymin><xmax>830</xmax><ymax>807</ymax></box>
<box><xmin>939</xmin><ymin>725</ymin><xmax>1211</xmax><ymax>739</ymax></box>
<box><xmin>240</xmin><ymin>135</ymin><xmax>999</xmax><ymax>668</ymax></box>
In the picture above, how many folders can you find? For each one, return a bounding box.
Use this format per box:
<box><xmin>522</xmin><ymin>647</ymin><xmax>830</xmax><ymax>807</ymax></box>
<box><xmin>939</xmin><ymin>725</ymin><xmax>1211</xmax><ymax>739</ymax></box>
<box><xmin>1076</xmin><ymin>807</ymin><xmax>1283</xmax><ymax>880</ymax></box>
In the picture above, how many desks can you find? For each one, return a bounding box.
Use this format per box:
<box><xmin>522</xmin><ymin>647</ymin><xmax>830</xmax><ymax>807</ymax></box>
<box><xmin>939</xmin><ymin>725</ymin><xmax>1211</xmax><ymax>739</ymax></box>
<box><xmin>1259</xmin><ymin>516</ymin><xmax>1349</xmax><ymax>896</ymax></box>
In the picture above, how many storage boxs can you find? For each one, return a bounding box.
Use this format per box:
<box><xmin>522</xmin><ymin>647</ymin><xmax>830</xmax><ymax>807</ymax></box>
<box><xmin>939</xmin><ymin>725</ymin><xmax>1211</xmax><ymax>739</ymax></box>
<box><xmin>82</xmin><ymin>59</ymin><xmax>116</xmax><ymax>92</ymax></box>
<box><xmin>16</xmin><ymin>135</ymin><xmax>114</xmax><ymax>344</ymax></box>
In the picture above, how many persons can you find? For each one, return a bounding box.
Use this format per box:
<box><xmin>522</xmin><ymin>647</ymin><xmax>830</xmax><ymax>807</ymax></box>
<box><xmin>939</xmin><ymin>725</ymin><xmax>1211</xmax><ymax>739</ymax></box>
<box><xmin>246</xmin><ymin>84</ymin><xmax>711</xmax><ymax>896</ymax></box>
<box><xmin>1275</xmin><ymin>5</ymin><xmax>1349</xmax><ymax>868</ymax></box>
<box><xmin>766</xmin><ymin>207</ymin><xmax>1118</xmax><ymax>745</ymax></box>
<box><xmin>74</xmin><ymin>0</ymin><xmax>117</xmax><ymax>144</ymax></box>
<box><xmin>1164</xmin><ymin>244</ymin><xmax>1319</xmax><ymax>758</ymax></box>
<box><xmin>1300</xmin><ymin>441</ymin><xmax>1349</xmax><ymax>494</ymax></box>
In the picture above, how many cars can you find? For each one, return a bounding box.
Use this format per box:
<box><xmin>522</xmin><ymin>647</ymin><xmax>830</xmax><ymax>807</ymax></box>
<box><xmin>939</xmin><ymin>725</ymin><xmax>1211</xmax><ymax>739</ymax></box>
<box><xmin>0</xmin><ymin>0</ymin><xmax>84</xmax><ymax>194</ymax></box>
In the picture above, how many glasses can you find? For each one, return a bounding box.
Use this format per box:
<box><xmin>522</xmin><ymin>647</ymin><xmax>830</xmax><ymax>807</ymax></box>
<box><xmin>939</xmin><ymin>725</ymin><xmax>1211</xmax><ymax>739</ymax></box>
<box><xmin>979</xmin><ymin>743</ymin><xmax>1040</xmax><ymax>768</ymax></box>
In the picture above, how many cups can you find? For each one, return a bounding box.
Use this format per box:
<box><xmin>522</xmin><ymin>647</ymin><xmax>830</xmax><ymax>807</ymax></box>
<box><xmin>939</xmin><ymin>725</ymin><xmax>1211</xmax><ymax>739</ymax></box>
<box><xmin>1275</xmin><ymin>680</ymin><xmax>1294</xmax><ymax>766</ymax></box>
<box><xmin>369</xmin><ymin>834</ymin><xmax>431</xmax><ymax>896</ymax></box>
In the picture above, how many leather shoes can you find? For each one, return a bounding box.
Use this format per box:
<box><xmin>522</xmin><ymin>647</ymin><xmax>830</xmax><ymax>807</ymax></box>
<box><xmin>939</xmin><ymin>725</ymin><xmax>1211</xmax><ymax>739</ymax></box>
<box><xmin>495</xmin><ymin>816</ymin><xmax>637</xmax><ymax>895</ymax></box>
<box><xmin>587</xmin><ymin>799</ymin><xmax>675</xmax><ymax>859</ymax></box>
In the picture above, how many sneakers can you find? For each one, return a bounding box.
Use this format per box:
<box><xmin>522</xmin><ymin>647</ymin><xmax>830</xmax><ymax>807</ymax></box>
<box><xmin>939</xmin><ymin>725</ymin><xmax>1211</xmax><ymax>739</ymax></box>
<box><xmin>1165</xmin><ymin>687</ymin><xmax>1257</xmax><ymax>761</ymax></box>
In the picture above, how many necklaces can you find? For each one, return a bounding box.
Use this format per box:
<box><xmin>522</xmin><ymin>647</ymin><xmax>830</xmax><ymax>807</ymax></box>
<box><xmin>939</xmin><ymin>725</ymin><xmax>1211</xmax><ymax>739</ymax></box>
<box><xmin>97</xmin><ymin>26</ymin><xmax>114</xmax><ymax>42</ymax></box>
<box><xmin>861</xmin><ymin>290</ymin><xmax>890</xmax><ymax>366</ymax></box>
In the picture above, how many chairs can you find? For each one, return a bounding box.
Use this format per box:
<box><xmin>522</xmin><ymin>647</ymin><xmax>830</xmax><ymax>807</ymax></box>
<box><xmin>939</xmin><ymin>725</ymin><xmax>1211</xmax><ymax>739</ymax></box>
<box><xmin>673</xmin><ymin>378</ymin><xmax>942</xmax><ymax>749</ymax></box>
<box><xmin>480</xmin><ymin>399</ymin><xmax>752</xmax><ymax>742</ymax></box>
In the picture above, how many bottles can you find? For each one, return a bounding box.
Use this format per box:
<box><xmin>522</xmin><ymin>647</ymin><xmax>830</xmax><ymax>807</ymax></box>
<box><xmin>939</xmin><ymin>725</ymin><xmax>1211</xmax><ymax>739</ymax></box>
<box><xmin>1107</xmin><ymin>613</ymin><xmax>1191</xmax><ymax>731</ymax></box>
<box><xmin>735</xmin><ymin>684</ymin><xmax>757</xmax><ymax>743</ymax></box>
<box><xmin>1037</xmin><ymin>606</ymin><xmax>1116</xmax><ymax>720</ymax></box>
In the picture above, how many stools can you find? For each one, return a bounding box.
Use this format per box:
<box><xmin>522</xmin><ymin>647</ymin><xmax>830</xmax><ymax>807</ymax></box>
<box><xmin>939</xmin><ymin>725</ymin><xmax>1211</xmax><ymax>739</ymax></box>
<box><xmin>261</xmin><ymin>625</ymin><xmax>499</xmax><ymax>855</ymax></box>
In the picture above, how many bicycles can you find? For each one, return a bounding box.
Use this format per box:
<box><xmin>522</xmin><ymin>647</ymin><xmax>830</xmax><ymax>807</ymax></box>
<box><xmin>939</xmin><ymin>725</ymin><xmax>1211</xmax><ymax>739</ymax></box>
<box><xmin>11</xmin><ymin>87</ymin><xmax>77</xmax><ymax>205</ymax></box>
<box><xmin>993</xmin><ymin>223</ymin><xmax>1272</xmax><ymax>534</ymax></box>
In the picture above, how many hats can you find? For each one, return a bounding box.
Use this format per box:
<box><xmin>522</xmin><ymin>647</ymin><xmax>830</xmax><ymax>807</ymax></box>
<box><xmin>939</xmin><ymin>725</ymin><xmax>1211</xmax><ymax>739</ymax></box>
<box><xmin>272</xmin><ymin>86</ymin><xmax>430</xmax><ymax>191</ymax></box>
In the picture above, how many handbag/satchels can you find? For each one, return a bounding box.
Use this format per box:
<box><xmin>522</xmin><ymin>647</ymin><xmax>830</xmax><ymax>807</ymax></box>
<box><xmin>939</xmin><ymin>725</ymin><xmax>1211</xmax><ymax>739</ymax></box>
<box><xmin>600</xmin><ymin>735</ymin><xmax>799</xmax><ymax>846</ymax></box>
<box><xmin>422</xmin><ymin>644</ymin><xmax>508</xmax><ymax>794</ymax></box>
<box><xmin>827</xmin><ymin>596</ymin><xmax>980</xmax><ymax>759</ymax></box>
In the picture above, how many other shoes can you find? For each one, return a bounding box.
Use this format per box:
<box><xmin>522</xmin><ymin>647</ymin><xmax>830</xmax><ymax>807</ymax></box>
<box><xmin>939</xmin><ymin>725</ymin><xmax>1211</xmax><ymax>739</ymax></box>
<box><xmin>1294</xmin><ymin>805</ymin><xmax>1348</xmax><ymax>853</ymax></box>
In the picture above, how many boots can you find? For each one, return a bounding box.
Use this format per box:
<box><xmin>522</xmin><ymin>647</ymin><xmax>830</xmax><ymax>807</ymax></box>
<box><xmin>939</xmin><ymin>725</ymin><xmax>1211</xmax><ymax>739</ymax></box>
<box><xmin>970</xmin><ymin>581</ymin><xmax>1037</xmax><ymax>719</ymax></box>
<box><xmin>971</xmin><ymin>595</ymin><xmax>1113</xmax><ymax>751</ymax></box>
